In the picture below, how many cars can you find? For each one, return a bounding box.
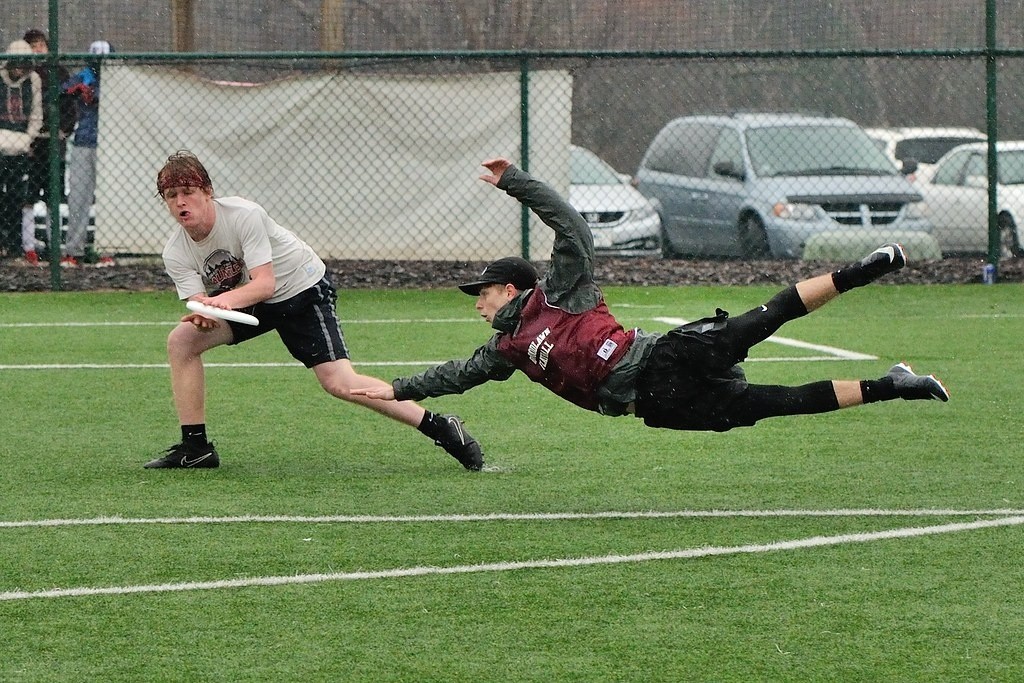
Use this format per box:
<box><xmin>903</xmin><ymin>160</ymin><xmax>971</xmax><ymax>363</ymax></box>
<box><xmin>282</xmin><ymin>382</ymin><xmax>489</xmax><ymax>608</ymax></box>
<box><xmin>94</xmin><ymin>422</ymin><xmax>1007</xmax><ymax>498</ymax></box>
<box><xmin>22</xmin><ymin>124</ymin><xmax>97</xmax><ymax>258</ymax></box>
<box><xmin>910</xmin><ymin>141</ymin><xmax>1024</xmax><ymax>266</ymax></box>
<box><xmin>864</xmin><ymin>124</ymin><xmax>989</xmax><ymax>188</ymax></box>
<box><xmin>564</xmin><ymin>143</ymin><xmax>664</xmax><ymax>260</ymax></box>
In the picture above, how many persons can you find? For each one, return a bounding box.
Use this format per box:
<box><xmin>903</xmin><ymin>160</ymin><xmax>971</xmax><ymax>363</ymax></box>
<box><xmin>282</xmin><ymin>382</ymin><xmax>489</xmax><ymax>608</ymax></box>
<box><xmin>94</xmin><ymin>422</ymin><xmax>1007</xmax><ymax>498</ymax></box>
<box><xmin>142</xmin><ymin>150</ymin><xmax>485</xmax><ymax>473</ymax></box>
<box><xmin>0</xmin><ymin>39</ymin><xmax>42</xmax><ymax>265</ymax></box>
<box><xmin>23</xmin><ymin>29</ymin><xmax>77</xmax><ymax>267</ymax></box>
<box><xmin>59</xmin><ymin>40</ymin><xmax>118</xmax><ymax>267</ymax></box>
<box><xmin>350</xmin><ymin>157</ymin><xmax>950</xmax><ymax>433</ymax></box>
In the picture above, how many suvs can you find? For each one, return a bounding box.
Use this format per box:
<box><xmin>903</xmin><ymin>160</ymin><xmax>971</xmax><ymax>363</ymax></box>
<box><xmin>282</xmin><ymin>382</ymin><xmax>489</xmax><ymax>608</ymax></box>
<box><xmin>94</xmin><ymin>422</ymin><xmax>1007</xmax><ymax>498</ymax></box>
<box><xmin>634</xmin><ymin>109</ymin><xmax>937</xmax><ymax>262</ymax></box>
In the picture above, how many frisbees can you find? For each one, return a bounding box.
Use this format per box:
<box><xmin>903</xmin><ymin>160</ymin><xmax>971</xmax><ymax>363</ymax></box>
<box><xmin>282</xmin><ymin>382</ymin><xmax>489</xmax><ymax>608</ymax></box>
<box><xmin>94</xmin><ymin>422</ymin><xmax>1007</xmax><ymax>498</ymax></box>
<box><xmin>185</xmin><ymin>299</ymin><xmax>260</xmax><ymax>327</ymax></box>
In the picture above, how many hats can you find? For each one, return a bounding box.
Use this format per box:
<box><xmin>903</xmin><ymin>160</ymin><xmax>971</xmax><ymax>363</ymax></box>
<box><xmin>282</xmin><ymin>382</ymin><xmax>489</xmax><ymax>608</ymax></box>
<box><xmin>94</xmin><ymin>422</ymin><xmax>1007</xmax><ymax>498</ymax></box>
<box><xmin>89</xmin><ymin>40</ymin><xmax>112</xmax><ymax>55</ymax></box>
<box><xmin>458</xmin><ymin>257</ymin><xmax>539</xmax><ymax>296</ymax></box>
<box><xmin>5</xmin><ymin>39</ymin><xmax>33</xmax><ymax>54</ymax></box>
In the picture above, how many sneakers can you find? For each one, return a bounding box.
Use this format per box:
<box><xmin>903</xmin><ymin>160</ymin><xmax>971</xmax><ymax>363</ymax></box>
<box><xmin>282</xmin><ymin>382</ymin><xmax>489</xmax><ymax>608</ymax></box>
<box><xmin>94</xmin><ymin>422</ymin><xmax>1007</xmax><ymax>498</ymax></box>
<box><xmin>89</xmin><ymin>258</ymin><xmax>115</xmax><ymax>269</ymax></box>
<box><xmin>143</xmin><ymin>439</ymin><xmax>220</xmax><ymax>468</ymax></box>
<box><xmin>887</xmin><ymin>361</ymin><xmax>950</xmax><ymax>402</ymax></box>
<box><xmin>59</xmin><ymin>257</ymin><xmax>83</xmax><ymax>271</ymax></box>
<box><xmin>25</xmin><ymin>251</ymin><xmax>38</xmax><ymax>265</ymax></box>
<box><xmin>435</xmin><ymin>414</ymin><xmax>485</xmax><ymax>473</ymax></box>
<box><xmin>844</xmin><ymin>242</ymin><xmax>907</xmax><ymax>287</ymax></box>
